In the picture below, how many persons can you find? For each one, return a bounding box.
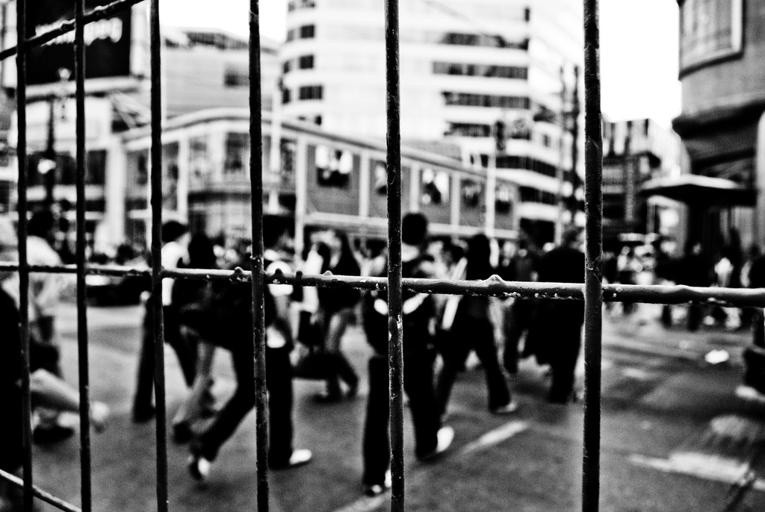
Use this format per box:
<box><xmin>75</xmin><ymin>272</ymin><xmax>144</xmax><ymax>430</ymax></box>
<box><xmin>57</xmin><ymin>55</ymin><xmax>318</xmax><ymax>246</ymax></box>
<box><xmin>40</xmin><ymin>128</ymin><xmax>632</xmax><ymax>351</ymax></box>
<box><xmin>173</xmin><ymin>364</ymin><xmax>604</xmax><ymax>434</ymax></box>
<box><xmin>600</xmin><ymin>220</ymin><xmax>764</xmax><ymax>333</ymax></box>
<box><xmin>131</xmin><ymin>211</ymin><xmax>588</xmax><ymax>495</ymax></box>
<box><xmin>1</xmin><ymin>212</ymin><xmax>115</xmax><ymax>495</ymax></box>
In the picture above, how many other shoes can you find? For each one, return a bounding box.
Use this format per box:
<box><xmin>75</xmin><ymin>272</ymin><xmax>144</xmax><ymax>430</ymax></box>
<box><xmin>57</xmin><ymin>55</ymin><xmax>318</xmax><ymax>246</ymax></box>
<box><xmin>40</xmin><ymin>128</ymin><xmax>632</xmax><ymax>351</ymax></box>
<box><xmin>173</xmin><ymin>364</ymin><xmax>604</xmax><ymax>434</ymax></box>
<box><xmin>268</xmin><ymin>448</ymin><xmax>313</xmax><ymax>472</ymax></box>
<box><xmin>435</xmin><ymin>426</ymin><xmax>455</xmax><ymax>452</ymax></box>
<box><xmin>187</xmin><ymin>438</ymin><xmax>211</xmax><ymax>481</ymax></box>
<box><xmin>491</xmin><ymin>399</ymin><xmax>517</xmax><ymax>413</ymax></box>
<box><xmin>364</xmin><ymin>470</ymin><xmax>391</xmax><ymax>497</ymax></box>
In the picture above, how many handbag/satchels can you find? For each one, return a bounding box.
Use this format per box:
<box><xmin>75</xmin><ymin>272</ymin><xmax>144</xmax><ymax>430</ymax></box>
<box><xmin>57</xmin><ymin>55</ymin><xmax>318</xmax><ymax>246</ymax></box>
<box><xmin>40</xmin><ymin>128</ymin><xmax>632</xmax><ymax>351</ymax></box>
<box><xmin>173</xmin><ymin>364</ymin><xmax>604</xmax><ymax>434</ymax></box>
<box><xmin>286</xmin><ymin>337</ymin><xmax>331</xmax><ymax>379</ymax></box>
